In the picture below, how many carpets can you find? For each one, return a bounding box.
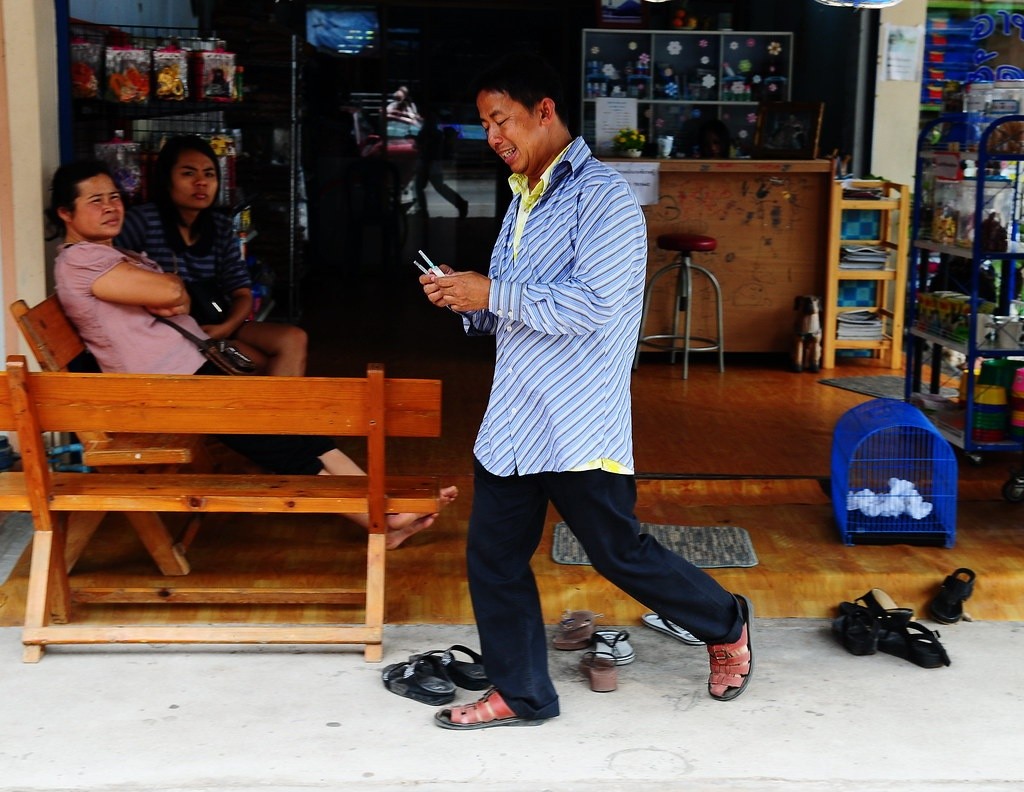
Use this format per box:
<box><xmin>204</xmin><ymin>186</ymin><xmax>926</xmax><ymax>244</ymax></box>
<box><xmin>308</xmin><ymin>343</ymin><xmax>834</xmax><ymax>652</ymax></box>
<box><xmin>551</xmin><ymin>519</ymin><xmax>759</xmax><ymax>572</ymax></box>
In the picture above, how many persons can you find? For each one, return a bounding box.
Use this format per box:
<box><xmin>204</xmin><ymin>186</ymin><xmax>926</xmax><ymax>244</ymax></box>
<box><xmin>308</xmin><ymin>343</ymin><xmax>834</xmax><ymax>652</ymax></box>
<box><xmin>405</xmin><ymin>112</ymin><xmax>468</xmax><ymax>222</ymax></box>
<box><xmin>43</xmin><ymin>153</ymin><xmax>459</xmax><ymax>552</ymax></box>
<box><xmin>436</xmin><ymin>56</ymin><xmax>757</xmax><ymax>731</ymax></box>
<box><xmin>697</xmin><ymin>118</ymin><xmax>732</xmax><ymax>159</ymax></box>
<box><xmin>113</xmin><ymin>133</ymin><xmax>308</xmax><ymax>377</ymax></box>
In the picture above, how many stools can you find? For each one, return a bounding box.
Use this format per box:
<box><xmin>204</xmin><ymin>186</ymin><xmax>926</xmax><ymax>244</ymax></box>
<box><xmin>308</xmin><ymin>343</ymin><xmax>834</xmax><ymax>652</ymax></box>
<box><xmin>638</xmin><ymin>233</ymin><xmax>728</xmax><ymax>378</ymax></box>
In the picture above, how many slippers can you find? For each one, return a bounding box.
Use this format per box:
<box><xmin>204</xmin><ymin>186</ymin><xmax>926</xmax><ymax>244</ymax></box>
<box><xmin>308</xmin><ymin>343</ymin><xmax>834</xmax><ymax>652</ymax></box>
<box><xmin>553</xmin><ymin>610</ymin><xmax>593</xmax><ymax>651</ymax></box>
<box><xmin>930</xmin><ymin>568</ymin><xmax>976</xmax><ymax>624</ymax></box>
<box><xmin>872</xmin><ymin>620</ymin><xmax>951</xmax><ymax>669</ymax></box>
<box><xmin>832</xmin><ymin>612</ymin><xmax>880</xmax><ymax>656</ymax></box>
<box><xmin>577</xmin><ymin>652</ymin><xmax>618</xmax><ymax>694</ymax></box>
<box><xmin>838</xmin><ymin>588</ymin><xmax>913</xmax><ymax>630</ymax></box>
<box><xmin>593</xmin><ymin>630</ymin><xmax>636</xmax><ymax>666</ymax></box>
<box><xmin>380</xmin><ymin>662</ymin><xmax>456</xmax><ymax>707</ymax></box>
<box><xmin>641</xmin><ymin>613</ymin><xmax>707</xmax><ymax>646</ymax></box>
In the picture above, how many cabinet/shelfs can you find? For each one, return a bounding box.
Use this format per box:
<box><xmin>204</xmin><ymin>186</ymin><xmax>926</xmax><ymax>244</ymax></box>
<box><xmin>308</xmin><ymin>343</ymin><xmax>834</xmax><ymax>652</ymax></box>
<box><xmin>578</xmin><ymin>30</ymin><xmax>796</xmax><ymax>157</ymax></box>
<box><xmin>907</xmin><ymin>115</ymin><xmax>1024</xmax><ymax>454</ymax></box>
<box><xmin>823</xmin><ymin>175</ymin><xmax>907</xmax><ymax>369</ymax></box>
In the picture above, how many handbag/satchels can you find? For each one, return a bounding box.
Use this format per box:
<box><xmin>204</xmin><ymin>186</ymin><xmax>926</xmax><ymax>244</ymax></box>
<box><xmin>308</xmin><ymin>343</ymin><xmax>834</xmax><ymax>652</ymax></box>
<box><xmin>200</xmin><ymin>337</ymin><xmax>258</xmax><ymax>377</ymax></box>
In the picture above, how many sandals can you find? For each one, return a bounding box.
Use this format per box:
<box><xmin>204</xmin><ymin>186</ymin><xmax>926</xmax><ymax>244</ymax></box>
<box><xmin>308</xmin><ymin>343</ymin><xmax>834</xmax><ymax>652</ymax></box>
<box><xmin>706</xmin><ymin>593</ymin><xmax>756</xmax><ymax>701</ymax></box>
<box><xmin>435</xmin><ymin>688</ymin><xmax>548</xmax><ymax>730</ymax></box>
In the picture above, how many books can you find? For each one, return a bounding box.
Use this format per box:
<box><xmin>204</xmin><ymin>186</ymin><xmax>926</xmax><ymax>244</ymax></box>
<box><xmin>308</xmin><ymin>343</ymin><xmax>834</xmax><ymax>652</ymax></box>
<box><xmin>840</xmin><ymin>242</ymin><xmax>892</xmax><ymax>271</ymax></box>
<box><xmin>837</xmin><ymin>310</ymin><xmax>886</xmax><ymax>343</ymax></box>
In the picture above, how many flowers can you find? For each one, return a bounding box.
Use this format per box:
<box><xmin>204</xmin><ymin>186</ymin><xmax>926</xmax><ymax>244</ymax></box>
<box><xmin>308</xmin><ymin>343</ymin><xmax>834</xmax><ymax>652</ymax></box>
<box><xmin>607</xmin><ymin>127</ymin><xmax>650</xmax><ymax>147</ymax></box>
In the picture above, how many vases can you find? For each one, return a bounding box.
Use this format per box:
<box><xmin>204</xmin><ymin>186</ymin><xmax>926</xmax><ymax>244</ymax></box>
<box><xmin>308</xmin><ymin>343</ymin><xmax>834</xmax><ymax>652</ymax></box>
<box><xmin>612</xmin><ymin>148</ymin><xmax>645</xmax><ymax>163</ymax></box>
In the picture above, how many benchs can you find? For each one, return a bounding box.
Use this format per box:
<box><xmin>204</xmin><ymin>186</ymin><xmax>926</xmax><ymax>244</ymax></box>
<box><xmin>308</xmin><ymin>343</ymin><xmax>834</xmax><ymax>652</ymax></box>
<box><xmin>11</xmin><ymin>293</ymin><xmax>196</xmax><ymax>578</ymax></box>
<box><xmin>0</xmin><ymin>356</ymin><xmax>441</xmax><ymax>654</ymax></box>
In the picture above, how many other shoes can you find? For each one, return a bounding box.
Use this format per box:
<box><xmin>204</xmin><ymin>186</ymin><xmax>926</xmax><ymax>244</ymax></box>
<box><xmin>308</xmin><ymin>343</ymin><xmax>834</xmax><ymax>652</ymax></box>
<box><xmin>408</xmin><ymin>644</ymin><xmax>491</xmax><ymax>690</ymax></box>
